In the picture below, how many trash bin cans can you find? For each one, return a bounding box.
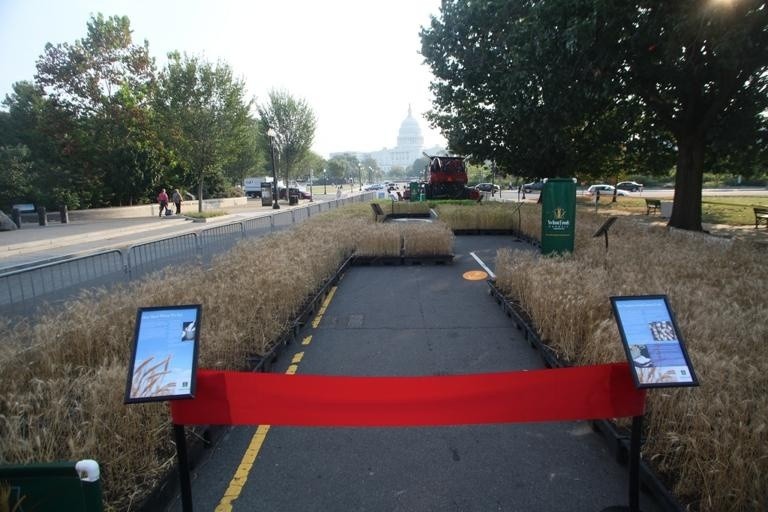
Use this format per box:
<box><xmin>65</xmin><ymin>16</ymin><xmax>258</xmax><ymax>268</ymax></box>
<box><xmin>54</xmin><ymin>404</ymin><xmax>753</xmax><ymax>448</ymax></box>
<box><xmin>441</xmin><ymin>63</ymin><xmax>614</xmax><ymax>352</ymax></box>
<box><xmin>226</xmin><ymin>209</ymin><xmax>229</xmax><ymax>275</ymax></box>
<box><xmin>259</xmin><ymin>180</ymin><xmax>273</xmax><ymax>207</ymax></box>
<box><xmin>660</xmin><ymin>202</ymin><xmax>673</xmax><ymax>218</ymax></box>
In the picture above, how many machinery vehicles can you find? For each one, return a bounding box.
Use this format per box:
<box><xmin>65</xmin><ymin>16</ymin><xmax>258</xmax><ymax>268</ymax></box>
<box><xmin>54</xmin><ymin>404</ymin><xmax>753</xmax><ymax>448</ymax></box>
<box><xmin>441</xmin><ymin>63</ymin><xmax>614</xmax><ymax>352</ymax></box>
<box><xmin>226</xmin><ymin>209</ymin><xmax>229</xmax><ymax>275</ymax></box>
<box><xmin>424</xmin><ymin>156</ymin><xmax>480</xmax><ymax>199</ymax></box>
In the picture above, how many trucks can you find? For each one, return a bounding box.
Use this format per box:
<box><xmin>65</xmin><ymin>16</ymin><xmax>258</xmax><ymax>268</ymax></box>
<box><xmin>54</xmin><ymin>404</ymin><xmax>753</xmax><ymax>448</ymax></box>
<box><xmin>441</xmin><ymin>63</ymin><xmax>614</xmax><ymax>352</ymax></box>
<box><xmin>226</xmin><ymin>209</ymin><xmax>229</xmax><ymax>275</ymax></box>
<box><xmin>244</xmin><ymin>177</ymin><xmax>303</xmax><ymax>198</ymax></box>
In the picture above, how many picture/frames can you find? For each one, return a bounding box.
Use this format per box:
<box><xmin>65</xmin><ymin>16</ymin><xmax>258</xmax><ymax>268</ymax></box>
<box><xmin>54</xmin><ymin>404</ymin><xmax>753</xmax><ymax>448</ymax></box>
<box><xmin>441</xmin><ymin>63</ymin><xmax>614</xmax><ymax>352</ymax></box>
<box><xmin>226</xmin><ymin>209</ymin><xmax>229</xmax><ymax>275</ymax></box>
<box><xmin>610</xmin><ymin>294</ymin><xmax>700</xmax><ymax>388</ymax></box>
<box><xmin>123</xmin><ymin>303</ymin><xmax>203</xmax><ymax>403</ymax></box>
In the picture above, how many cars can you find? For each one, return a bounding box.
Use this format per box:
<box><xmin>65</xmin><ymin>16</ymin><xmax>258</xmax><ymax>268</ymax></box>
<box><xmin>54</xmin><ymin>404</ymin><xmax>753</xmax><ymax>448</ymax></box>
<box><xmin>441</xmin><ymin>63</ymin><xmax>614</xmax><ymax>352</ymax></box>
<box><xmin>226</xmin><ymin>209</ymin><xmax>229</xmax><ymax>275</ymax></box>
<box><xmin>522</xmin><ymin>180</ymin><xmax>548</xmax><ymax>193</ymax></box>
<box><xmin>366</xmin><ymin>180</ymin><xmax>422</xmax><ymax>200</ymax></box>
<box><xmin>475</xmin><ymin>183</ymin><xmax>500</xmax><ymax>193</ymax></box>
<box><xmin>586</xmin><ymin>181</ymin><xmax>644</xmax><ymax>197</ymax></box>
<box><xmin>274</xmin><ymin>187</ymin><xmax>313</xmax><ymax>201</ymax></box>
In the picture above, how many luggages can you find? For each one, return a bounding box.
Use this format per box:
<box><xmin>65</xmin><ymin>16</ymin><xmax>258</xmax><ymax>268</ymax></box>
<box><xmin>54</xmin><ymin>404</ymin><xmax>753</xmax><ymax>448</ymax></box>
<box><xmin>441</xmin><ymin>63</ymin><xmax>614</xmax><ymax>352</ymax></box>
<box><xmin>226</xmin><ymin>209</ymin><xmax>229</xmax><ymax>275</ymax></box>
<box><xmin>166</xmin><ymin>210</ymin><xmax>173</xmax><ymax>216</ymax></box>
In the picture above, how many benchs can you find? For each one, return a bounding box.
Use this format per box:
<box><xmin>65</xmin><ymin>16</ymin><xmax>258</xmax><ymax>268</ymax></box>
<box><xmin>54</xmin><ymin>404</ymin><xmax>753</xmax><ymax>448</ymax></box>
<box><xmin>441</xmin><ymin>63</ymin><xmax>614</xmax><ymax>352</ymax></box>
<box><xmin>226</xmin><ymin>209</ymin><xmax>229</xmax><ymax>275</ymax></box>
<box><xmin>753</xmin><ymin>208</ymin><xmax>768</xmax><ymax>229</ymax></box>
<box><xmin>646</xmin><ymin>199</ymin><xmax>661</xmax><ymax>215</ymax></box>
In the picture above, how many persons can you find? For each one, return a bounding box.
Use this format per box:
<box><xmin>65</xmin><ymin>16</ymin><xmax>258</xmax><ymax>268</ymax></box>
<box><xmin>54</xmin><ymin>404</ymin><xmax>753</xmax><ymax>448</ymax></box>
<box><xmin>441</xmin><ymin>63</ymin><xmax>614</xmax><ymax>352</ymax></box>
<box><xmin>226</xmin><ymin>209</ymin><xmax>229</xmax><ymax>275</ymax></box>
<box><xmin>172</xmin><ymin>188</ymin><xmax>184</xmax><ymax>214</ymax></box>
<box><xmin>595</xmin><ymin>187</ymin><xmax>600</xmax><ymax>201</ymax></box>
<box><xmin>506</xmin><ymin>180</ymin><xmax>513</xmax><ymax>190</ymax></box>
<box><xmin>335</xmin><ymin>186</ymin><xmax>341</xmax><ymax>199</ymax></box>
<box><xmin>156</xmin><ymin>188</ymin><xmax>169</xmax><ymax>217</ymax></box>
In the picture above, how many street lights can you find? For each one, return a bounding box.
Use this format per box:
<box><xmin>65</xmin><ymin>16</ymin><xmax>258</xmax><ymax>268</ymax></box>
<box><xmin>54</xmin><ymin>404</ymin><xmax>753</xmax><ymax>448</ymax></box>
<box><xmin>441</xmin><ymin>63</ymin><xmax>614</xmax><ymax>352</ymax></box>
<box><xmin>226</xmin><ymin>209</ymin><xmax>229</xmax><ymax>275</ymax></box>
<box><xmin>266</xmin><ymin>124</ymin><xmax>281</xmax><ymax>210</ymax></box>
<box><xmin>368</xmin><ymin>166</ymin><xmax>374</xmax><ymax>182</ymax></box>
<box><xmin>356</xmin><ymin>162</ymin><xmax>362</xmax><ymax>190</ymax></box>
<box><xmin>322</xmin><ymin>168</ymin><xmax>327</xmax><ymax>194</ymax></box>
